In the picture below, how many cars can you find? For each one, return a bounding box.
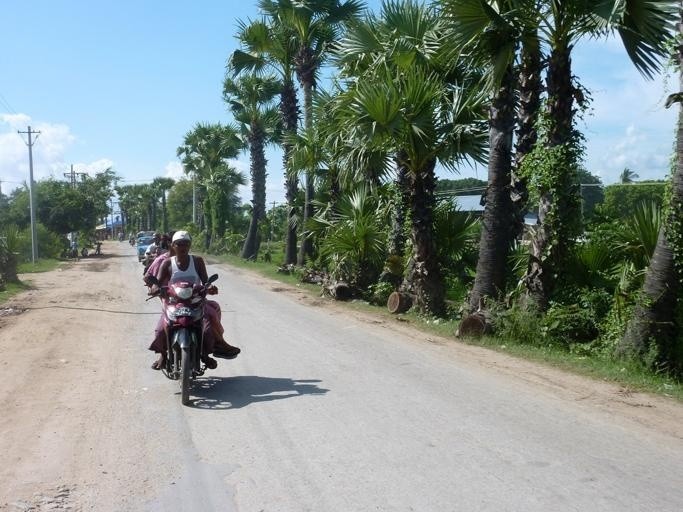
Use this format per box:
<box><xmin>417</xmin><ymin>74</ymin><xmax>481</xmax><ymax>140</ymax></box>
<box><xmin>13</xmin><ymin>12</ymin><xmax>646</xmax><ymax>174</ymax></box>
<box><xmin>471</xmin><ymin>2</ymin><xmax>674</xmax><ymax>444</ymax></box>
<box><xmin>136</xmin><ymin>230</ymin><xmax>156</xmax><ymax>237</ymax></box>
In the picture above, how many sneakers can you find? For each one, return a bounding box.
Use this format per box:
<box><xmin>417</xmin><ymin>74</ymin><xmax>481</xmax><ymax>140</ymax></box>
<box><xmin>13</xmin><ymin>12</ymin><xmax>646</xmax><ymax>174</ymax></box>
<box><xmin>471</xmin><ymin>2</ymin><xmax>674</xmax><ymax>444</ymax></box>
<box><xmin>213</xmin><ymin>339</ymin><xmax>241</xmax><ymax>359</ymax></box>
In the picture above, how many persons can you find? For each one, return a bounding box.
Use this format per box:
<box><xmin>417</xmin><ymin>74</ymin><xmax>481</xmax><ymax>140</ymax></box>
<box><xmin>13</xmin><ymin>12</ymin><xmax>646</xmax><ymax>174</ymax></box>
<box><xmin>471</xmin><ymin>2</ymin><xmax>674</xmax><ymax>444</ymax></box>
<box><xmin>61</xmin><ymin>231</ymin><xmax>102</xmax><ymax>257</ymax></box>
<box><xmin>142</xmin><ymin>229</ymin><xmax>241</xmax><ymax>370</ymax></box>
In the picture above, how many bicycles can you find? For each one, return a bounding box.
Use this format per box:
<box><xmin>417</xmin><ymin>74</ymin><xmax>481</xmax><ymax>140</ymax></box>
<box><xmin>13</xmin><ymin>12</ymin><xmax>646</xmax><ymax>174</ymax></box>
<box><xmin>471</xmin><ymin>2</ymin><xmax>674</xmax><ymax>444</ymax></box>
<box><xmin>95</xmin><ymin>242</ymin><xmax>103</xmax><ymax>256</ymax></box>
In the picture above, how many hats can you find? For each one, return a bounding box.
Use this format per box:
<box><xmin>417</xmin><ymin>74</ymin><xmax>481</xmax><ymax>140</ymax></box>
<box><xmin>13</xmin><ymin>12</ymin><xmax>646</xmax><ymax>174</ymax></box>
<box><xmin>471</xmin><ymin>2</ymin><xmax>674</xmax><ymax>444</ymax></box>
<box><xmin>152</xmin><ymin>230</ymin><xmax>193</xmax><ymax>248</ymax></box>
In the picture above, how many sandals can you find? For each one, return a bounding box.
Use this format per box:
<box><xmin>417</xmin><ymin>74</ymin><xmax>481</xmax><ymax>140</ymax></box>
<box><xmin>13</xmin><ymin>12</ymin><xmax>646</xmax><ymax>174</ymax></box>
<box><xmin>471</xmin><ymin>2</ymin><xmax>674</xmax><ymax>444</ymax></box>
<box><xmin>151</xmin><ymin>357</ymin><xmax>167</xmax><ymax>370</ymax></box>
<box><xmin>201</xmin><ymin>355</ymin><xmax>218</xmax><ymax>369</ymax></box>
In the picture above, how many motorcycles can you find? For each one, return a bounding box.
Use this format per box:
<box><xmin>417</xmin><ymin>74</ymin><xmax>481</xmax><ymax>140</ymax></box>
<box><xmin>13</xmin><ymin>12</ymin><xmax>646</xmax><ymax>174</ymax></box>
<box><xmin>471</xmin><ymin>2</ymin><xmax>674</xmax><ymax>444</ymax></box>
<box><xmin>118</xmin><ymin>236</ymin><xmax>219</xmax><ymax>410</ymax></box>
